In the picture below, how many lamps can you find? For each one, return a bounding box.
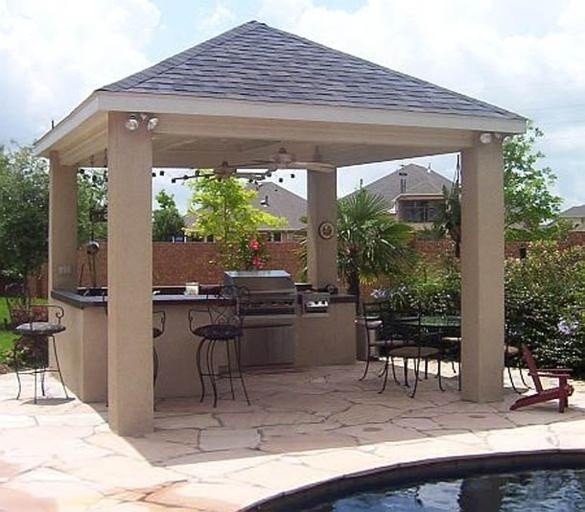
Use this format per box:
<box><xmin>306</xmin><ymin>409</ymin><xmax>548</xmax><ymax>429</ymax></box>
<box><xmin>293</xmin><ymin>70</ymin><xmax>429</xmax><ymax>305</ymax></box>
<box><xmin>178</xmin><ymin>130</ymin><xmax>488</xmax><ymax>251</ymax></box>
<box><xmin>479</xmin><ymin>132</ymin><xmax>503</xmax><ymax>146</ymax></box>
<box><xmin>125</xmin><ymin>112</ymin><xmax>159</xmax><ymax>132</ymax></box>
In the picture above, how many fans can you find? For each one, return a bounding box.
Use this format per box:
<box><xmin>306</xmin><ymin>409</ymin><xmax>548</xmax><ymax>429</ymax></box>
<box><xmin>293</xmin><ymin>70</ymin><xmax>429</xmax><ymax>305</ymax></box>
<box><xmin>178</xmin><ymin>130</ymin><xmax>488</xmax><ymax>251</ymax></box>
<box><xmin>244</xmin><ymin>148</ymin><xmax>336</xmax><ymax>175</ymax></box>
<box><xmin>176</xmin><ymin>162</ymin><xmax>264</xmax><ymax>179</ymax></box>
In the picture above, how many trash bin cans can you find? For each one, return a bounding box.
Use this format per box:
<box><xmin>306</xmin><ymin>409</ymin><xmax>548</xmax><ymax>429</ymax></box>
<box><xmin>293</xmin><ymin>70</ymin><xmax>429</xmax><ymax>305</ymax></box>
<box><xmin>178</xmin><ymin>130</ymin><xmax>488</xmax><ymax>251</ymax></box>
<box><xmin>353</xmin><ymin>316</ymin><xmax>384</xmax><ymax>361</ymax></box>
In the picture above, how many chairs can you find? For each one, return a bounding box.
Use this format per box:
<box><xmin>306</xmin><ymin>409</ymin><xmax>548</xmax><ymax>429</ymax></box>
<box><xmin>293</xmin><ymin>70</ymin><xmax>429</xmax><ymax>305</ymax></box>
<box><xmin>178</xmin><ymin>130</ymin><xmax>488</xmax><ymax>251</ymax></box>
<box><xmin>509</xmin><ymin>342</ymin><xmax>576</xmax><ymax>414</ymax></box>
<box><xmin>359</xmin><ymin>298</ymin><xmax>535</xmax><ymax>398</ymax></box>
<box><xmin>188</xmin><ymin>284</ymin><xmax>251</xmax><ymax>407</ymax></box>
<box><xmin>100</xmin><ymin>291</ymin><xmax>165</xmax><ymax>411</ymax></box>
<box><xmin>3</xmin><ymin>272</ymin><xmax>70</xmax><ymax>403</ymax></box>
